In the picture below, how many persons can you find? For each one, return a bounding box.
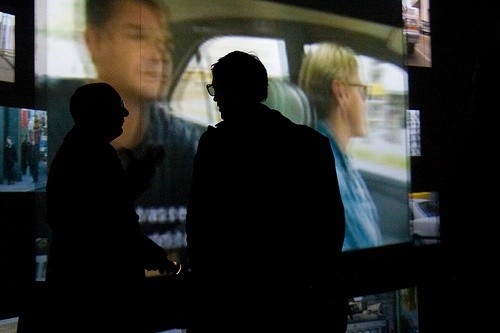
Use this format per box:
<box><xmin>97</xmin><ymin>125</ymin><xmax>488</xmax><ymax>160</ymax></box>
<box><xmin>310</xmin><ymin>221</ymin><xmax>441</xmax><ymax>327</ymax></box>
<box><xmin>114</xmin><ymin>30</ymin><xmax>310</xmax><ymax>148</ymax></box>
<box><xmin>297</xmin><ymin>40</ymin><xmax>384</xmax><ymax>252</ymax></box>
<box><xmin>187</xmin><ymin>51</ymin><xmax>345</xmax><ymax>333</ymax></box>
<box><xmin>45</xmin><ymin>83</ymin><xmax>180</xmax><ymax>333</ymax></box>
<box><xmin>34</xmin><ymin>0</ymin><xmax>211</xmax><ymax>196</ymax></box>
<box><xmin>3</xmin><ymin>135</ymin><xmax>41</xmax><ymax>185</ymax></box>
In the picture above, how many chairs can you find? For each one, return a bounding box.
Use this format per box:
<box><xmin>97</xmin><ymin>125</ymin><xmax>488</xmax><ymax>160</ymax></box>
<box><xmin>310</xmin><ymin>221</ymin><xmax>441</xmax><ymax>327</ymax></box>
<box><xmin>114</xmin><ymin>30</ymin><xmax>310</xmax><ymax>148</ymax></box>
<box><xmin>259</xmin><ymin>78</ymin><xmax>316</xmax><ymax>131</ymax></box>
